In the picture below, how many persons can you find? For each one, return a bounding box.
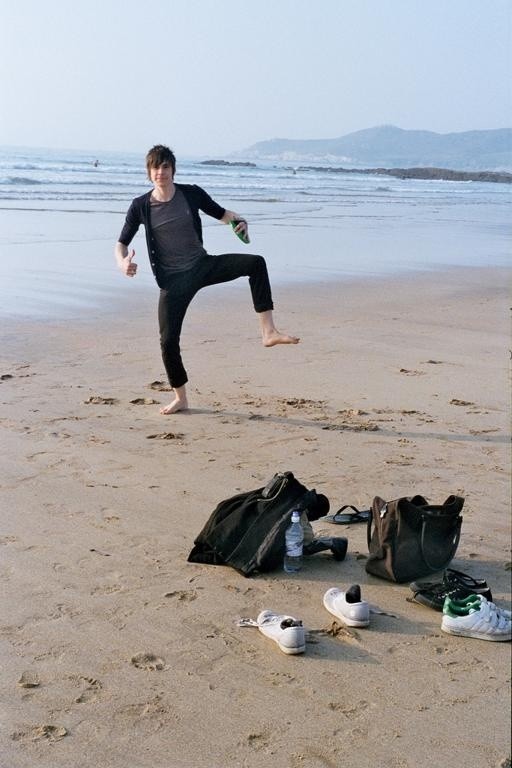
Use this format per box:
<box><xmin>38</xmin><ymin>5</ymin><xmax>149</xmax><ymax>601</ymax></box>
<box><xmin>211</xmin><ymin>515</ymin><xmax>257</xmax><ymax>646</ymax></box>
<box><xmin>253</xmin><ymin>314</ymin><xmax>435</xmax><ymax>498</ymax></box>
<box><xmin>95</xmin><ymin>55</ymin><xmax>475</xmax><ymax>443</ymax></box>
<box><xmin>117</xmin><ymin>146</ymin><xmax>300</xmax><ymax>414</ymax></box>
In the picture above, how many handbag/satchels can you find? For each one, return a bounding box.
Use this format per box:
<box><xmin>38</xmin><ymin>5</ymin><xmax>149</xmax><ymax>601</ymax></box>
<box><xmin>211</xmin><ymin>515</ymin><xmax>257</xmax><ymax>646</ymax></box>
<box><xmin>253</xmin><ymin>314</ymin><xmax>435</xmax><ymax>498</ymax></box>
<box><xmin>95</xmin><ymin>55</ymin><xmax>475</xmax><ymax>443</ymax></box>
<box><xmin>365</xmin><ymin>495</ymin><xmax>464</xmax><ymax>584</ymax></box>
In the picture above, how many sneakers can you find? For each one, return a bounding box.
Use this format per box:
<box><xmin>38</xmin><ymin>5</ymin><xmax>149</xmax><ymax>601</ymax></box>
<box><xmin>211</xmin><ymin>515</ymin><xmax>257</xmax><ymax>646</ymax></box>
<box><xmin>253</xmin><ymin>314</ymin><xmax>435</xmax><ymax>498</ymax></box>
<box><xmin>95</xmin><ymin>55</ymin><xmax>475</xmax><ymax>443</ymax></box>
<box><xmin>322</xmin><ymin>585</ymin><xmax>370</xmax><ymax>628</ymax></box>
<box><xmin>256</xmin><ymin>610</ymin><xmax>305</xmax><ymax>655</ymax></box>
<box><xmin>411</xmin><ymin>570</ymin><xmax>511</xmax><ymax>642</ymax></box>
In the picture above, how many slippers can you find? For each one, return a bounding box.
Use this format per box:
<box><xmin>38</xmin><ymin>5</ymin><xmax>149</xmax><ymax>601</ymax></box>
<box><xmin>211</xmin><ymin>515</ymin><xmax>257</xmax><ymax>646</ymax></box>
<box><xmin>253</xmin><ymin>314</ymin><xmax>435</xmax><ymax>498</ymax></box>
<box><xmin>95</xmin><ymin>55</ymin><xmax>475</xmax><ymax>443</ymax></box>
<box><xmin>320</xmin><ymin>505</ymin><xmax>369</xmax><ymax>525</ymax></box>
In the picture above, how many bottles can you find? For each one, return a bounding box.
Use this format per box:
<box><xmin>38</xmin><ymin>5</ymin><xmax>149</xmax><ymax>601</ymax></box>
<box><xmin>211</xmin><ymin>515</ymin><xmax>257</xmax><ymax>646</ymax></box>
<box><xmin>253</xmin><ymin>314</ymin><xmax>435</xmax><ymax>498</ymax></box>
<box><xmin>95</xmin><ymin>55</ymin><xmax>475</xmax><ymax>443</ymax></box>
<box><xmin>282</xmin><ymin>511</ymin><xmax>305</xmax><ymax>572</ymax></box>
